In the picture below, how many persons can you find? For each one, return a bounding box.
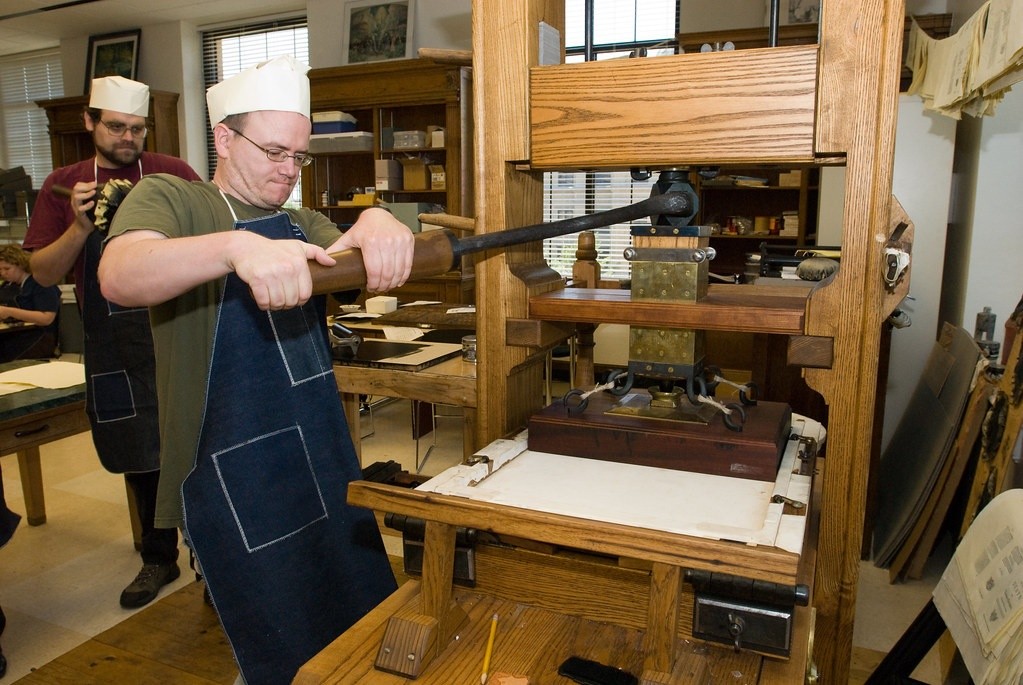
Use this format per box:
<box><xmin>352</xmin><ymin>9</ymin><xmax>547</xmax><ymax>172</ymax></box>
<box><xmin>20</xmin><ymin>75</ymin><xmax>204</xmax><ymax>609</ymax></box>
<box><xmin>96</xmin><ymin>59</ymin><xmax>415</xmax><ymax>685</ymax></box>
<box><xmin>0</xmin><ymin>243</ymin><xmax>63</xmax><ymax>364</ymax></box>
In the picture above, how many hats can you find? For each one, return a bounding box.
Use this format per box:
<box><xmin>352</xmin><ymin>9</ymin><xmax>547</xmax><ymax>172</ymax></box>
<box><xmin>206</xmin><ymin>58</ymin><xmax>311</xmax><ymax>134</ymax></box>
<box><xmin>89</xmin><ymin>76</ymin><xmax>150</xmax><ymax>116</ymax></box>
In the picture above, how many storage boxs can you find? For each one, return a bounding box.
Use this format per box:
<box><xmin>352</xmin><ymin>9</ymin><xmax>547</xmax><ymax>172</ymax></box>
<box><xmin>375</xmin><ymin>158</ymin><xmax>446</xmax><ymax>192</ymax></box>
<box><xmin>382</xmin><ymin>125</ymin><xmax>447</xmax><ymax>150</ymax></box>
<box><xmin>382</xmin><ymin>202</ymin><xmax>444</xmax><ymax>232</ymax></box>
<box><xmin>0</xmin><ymin>166</ymin><xmax>40</xmax><ymax>220</ymax></box>
<box><xmin>306</xmin><ymin>111</ymin><xmax>374</xmax><ymax>154</ymax></box>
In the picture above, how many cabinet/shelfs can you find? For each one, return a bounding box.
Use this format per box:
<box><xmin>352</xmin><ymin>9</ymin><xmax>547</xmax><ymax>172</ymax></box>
<box><xmin>35</xmin><ymin>88</ymin><xmax>180</xmax><ymax>171</ymax></box>
<box><xmin>300</xmin><ymin>57</ymin><xmax>476</xmax><ymax>316</ymax></box>
<box><xmin>676</xmin><ymin>12</ymin><xmax>953</xmax><ymax>286</ymax></box>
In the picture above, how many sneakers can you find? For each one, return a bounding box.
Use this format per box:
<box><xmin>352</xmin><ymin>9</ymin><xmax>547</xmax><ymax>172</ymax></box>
<box><xmin>120</xmin><ymin>564</ymin><xmax>181</xmax><ymax>610</ymax></box>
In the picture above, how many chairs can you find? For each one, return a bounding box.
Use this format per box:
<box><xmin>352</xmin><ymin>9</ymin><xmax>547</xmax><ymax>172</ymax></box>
<box><xmin>416</xmin><ymin>328</ymin><xmax>477</xmax><ymax>474</ymax></box>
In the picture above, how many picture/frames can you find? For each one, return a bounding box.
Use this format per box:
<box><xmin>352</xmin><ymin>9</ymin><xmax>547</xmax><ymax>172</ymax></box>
<box><xmin>343</xmin><ymin>0</ymin><xmax>415</xmax><ymax>65</ymax></box>
<box><xmin>83</xmin><ymin>28</ymin><xmax>141</xmax><ymax>94</ymax></box>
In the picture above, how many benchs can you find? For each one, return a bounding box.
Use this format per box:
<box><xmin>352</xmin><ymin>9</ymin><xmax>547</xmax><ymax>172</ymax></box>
<box><xmin>0</xmin><ymin>359</ymin><xmax>143</xmax><ymax>553</ymax></box>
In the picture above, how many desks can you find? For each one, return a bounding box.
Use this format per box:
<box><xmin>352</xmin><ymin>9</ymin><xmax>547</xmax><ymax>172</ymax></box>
<box><xmin>328</xmin><ymin>299</ymin><xmax>480</xmax><ymax>469</ymax></box>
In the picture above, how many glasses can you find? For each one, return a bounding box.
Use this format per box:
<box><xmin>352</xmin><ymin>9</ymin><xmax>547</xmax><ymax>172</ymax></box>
<box><xmin>99</xmin><ymin>119</ymin><xmax>149</xmax><ymax>138</ymax></box>
<box><xmin>230</xmin><ymin>128</ymin><xmax>314</xmax><ymax>166</ymax></box>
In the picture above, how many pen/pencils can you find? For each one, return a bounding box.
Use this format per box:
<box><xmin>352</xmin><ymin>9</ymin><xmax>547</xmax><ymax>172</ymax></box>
<box><xmin>481</xmin><ymin>613</ymin><xmax>498</xmax><ymax>685</ymax></box>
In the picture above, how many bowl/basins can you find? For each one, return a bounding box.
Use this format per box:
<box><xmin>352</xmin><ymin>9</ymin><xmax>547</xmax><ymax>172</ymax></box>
<box><xmin>340</xmin><ymin>305</ymin><xmax>361</xmax><ymax>312</ymax></box>
